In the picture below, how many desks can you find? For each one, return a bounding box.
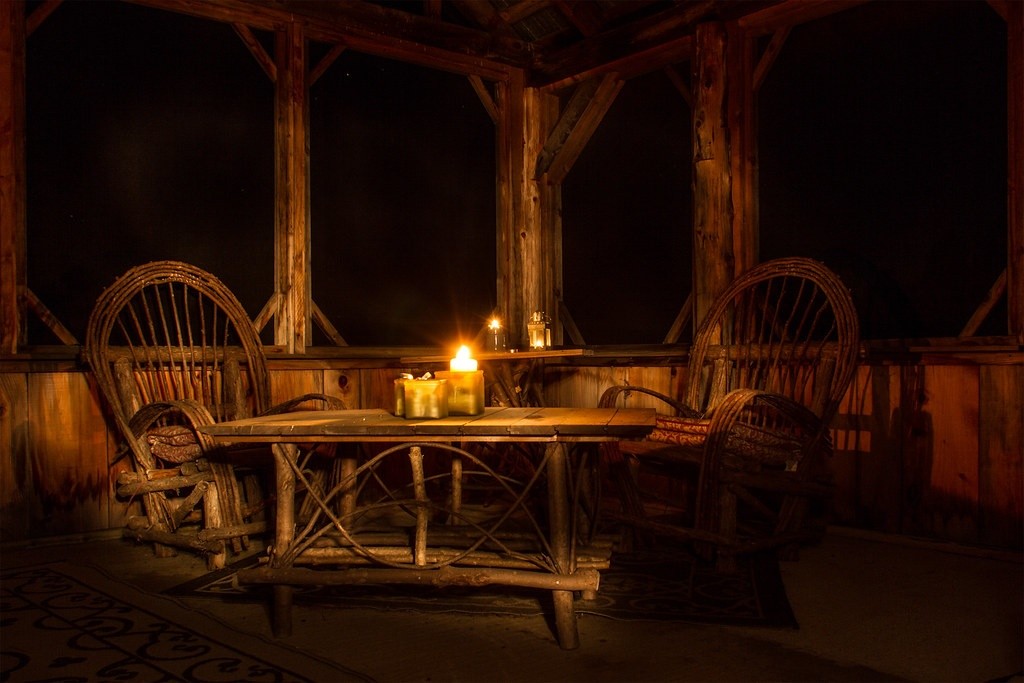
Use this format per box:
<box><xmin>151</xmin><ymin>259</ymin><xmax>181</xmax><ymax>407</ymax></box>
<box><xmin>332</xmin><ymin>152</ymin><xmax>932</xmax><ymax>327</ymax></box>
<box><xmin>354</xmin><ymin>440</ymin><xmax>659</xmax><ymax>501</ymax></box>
<box><xmin>193</xmin><ymin>408</ymin><xmax>657</xmax><ymax>652</ymax></box>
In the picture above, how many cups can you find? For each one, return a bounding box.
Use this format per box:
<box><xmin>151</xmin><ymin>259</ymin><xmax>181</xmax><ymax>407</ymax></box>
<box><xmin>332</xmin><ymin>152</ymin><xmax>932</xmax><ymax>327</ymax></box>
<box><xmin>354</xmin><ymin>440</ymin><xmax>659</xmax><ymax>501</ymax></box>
<box><xmin>486</xmin><ymin>326</ymin><xmax>508</xmax><ymax>352</ymax></box>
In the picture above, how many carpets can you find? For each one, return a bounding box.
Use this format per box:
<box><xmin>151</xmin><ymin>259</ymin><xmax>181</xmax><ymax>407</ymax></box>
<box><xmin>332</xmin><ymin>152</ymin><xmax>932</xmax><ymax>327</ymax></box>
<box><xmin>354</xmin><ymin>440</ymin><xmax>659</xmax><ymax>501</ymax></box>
<box><xmin>147</xmin><ymin>543</ymin><xmax>802</xmax><ymax>631</ymax></box>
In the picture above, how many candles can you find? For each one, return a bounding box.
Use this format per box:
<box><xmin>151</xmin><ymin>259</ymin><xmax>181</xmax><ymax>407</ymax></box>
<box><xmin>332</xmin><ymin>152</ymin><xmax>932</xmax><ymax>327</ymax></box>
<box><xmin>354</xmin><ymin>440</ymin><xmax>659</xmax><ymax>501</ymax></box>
<box><xmin>394</xmin><ymin>372</ymin><xmax>417</xmax><ymax>416</ymax></box>
<box><xmin>405</xmin><ymin>372</ymin><xmax>450</xmax><ymax>419</ymax></box>
<box><xmin>450</xmin><ymin>345</ymin><xmax>479</xmax><ymax>372</ymax></box>
<box><xmin>485</xmin><ymin>316</ymin><xmax>508</xmax><ymax>352</ymax></box>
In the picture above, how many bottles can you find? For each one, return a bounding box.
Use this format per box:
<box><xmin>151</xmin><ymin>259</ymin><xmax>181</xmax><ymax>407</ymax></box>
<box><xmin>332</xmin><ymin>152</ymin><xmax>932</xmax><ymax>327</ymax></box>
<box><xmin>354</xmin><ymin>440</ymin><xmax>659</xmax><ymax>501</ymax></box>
<box><xmin>527</xmin><ymin>311</ymin><xmax>552</xmax><ymax>351</ymax></box>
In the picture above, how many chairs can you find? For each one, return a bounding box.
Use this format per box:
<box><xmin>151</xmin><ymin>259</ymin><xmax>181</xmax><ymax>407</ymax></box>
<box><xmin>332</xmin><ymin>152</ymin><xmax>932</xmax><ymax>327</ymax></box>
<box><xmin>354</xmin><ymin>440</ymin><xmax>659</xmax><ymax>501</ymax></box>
<box><xmin>79</xmin><ymin>260</ymin><xmax>325</xmax><ymax>572</ymax></box>
<box><xmin>582</xmin><ymin>255</ymin><xmax>859</xmax><ymax>573</ymax></box>
